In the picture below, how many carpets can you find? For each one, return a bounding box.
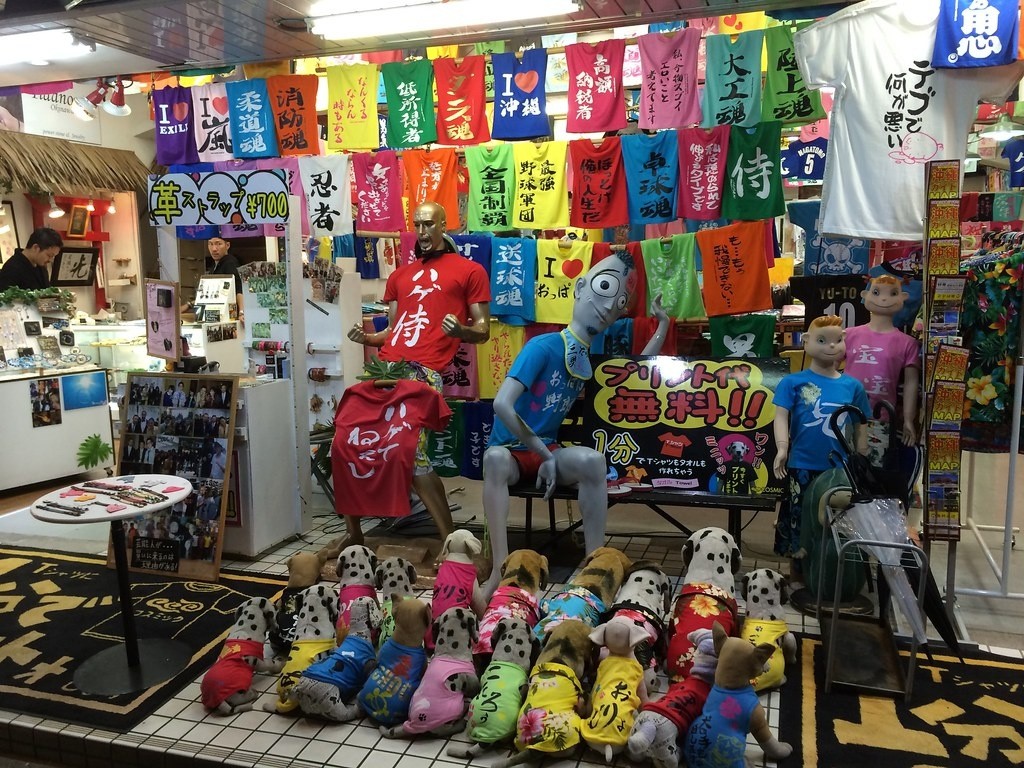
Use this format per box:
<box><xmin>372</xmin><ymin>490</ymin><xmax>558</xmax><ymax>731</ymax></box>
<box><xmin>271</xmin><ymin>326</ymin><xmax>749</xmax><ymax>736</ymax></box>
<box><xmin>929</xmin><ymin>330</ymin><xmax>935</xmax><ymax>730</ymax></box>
<box><xmin>0</xmin><ymin>545</ymin><xmax>289</xmax><ymax>735</ymax></box>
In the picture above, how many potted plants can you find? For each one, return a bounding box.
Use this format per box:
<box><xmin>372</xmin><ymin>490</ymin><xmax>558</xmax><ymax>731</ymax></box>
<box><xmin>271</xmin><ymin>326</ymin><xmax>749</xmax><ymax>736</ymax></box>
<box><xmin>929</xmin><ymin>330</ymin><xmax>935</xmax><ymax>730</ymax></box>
<box><xmin>75</xmin><ymin>432</ymin><xmax>113</xmax><ymax>480</ymax></box>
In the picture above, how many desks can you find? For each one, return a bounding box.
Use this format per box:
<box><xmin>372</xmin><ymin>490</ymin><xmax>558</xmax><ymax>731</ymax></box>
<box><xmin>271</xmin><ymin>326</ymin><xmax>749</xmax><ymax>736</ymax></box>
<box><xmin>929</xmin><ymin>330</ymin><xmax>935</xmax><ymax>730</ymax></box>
<box><xmin>29</xmin><ymin>474</ymin><xmax>194</xmax><ymax>696</ymax></box>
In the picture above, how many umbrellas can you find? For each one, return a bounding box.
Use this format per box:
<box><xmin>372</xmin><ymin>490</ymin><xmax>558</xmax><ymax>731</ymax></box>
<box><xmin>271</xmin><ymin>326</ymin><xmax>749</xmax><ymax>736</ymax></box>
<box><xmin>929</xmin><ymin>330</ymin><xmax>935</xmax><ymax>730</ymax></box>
<box><xmin>828</xmin><ymin>399</ymin><xmax>967</xmax><ymax>666</ymax></box>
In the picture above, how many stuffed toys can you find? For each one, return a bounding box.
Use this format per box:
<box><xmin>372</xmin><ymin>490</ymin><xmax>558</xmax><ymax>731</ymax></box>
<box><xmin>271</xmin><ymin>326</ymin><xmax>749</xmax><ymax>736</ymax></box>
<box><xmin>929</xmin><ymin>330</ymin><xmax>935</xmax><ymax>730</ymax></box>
<box><xmin>201</xmin><ymin>526</ymin><xmax>797</xmax><ymax>768</ymax></box>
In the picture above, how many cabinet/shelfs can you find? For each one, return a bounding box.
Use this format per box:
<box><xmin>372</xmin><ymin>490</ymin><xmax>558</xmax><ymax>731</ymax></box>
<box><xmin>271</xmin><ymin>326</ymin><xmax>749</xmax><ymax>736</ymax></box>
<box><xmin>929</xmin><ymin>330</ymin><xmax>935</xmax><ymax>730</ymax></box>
<box><xmin>70</xmin><ymin>322</ymin><xmax>249</xmax><ymax>441</ymax></box>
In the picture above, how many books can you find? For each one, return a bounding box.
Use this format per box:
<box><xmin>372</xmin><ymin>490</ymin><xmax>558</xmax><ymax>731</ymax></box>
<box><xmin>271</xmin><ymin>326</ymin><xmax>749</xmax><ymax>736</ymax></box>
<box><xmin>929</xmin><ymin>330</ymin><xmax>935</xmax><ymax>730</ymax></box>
<box><xmin>923</xmin><ymin>159</ymin><xmax>970</xmax><ymax>535</ymax></box>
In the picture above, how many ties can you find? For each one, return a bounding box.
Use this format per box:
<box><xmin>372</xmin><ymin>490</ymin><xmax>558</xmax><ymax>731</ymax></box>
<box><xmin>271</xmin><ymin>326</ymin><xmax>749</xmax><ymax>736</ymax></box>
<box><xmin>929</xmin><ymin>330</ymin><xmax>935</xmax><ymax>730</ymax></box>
<box><xmin>177</xmin><ymin>392</ymin><xmax>181</xmax><ymax>407</ymax></box>
<box><xmin>147</xmin><ymin>450</ymin><xmax>150</xmax><ymax>462</ymax></box>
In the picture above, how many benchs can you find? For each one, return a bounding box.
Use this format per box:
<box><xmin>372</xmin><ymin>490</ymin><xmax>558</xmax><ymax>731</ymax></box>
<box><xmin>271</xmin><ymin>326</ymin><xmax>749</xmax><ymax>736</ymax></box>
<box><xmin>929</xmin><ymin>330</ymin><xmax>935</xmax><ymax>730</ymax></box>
<box><xmin>517</xmin><ymin>480</ymin><xmax>771</xmax><ymax>554</ymax></box>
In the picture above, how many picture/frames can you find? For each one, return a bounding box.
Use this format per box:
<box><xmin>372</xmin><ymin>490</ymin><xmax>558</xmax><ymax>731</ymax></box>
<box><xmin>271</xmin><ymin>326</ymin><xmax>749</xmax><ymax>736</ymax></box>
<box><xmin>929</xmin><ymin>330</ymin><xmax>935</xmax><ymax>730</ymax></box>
<box><xmin>66</xmin><ymin>205</ymin><xmax>91</xmax><ymax>238</ymax></box>
<box><xmin>0</xmin><ymin>200</ymin><xmax>20</xmax><ymax>264</ymax></box>
<box><xmin>49</xmin><ymin>246</ymin><xmax>100</xmax><ymax>287</ymax></box>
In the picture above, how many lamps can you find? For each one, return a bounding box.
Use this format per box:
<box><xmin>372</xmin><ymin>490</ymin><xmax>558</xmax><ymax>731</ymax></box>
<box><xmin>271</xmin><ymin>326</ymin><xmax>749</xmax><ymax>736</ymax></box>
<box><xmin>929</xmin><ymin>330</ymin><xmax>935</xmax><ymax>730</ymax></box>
<box><xmin>48</xmin><ymin>195</ymin><xmax>65</xmax><ymax>219</ymax></box>
<box><xmin>69</xmin><ymin>84</ymin><xmax>109</xmax><ymax>121</ymax></box>
<box><xmin>101</xmin><ymin>81</ymin><xmax>131</xmax><ymax>117</ymax></box>
<box><xmin>86</xmin><ymin>200</ymin><xmax>96</xmax><ymax>211</ymax></box>
<box><xmin>108</xmin><ymin>200</ymin><xmax>116</xmax><ymax>215</ymax></box>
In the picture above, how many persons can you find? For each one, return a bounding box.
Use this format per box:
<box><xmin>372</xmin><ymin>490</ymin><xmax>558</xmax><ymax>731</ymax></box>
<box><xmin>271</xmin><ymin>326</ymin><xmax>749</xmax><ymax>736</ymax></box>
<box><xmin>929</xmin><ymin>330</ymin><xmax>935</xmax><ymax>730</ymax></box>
<box><xmin>303</xmin><ymin>258</ymin><xmax>341</xmax><ymax>303</ymax></box>
<box><xmin>180</xmin><ymin>238</ymin><xmax>245</xmax><ymax>328</ymax></box>
<box><xmin>834</xmin><ymin>275</ymin><xmax>920</xmax><ymax>448</ymax></box>
<box><xmin>121</xmin><ymin>384</ymin><xmax>232</xmax><ymax>561</ymax></box>
<box><xmin>313</xmin><ymin>202</ymin><xmax>491</xmax><ymax>570</ymax></box>
<box><xmin>479</xmin><ymin>249</ymin><xmax>670</xmax><ymax>605</ymax></box>
<box><xmin>0</xmin><ymin>228</ymin><xmax>63</xmax><ymax>293</ymax></box>
<box><xmin>240</xmin><ymin>262</ymin><xmax>289</xmax><ymax>337</ymax></box>
<box><xmin>772</xmin><ymin>316</ymin><xmax>873</xmax><ymax>591</ymax></box>
<box><xmin>30</xmin><ymin>379</ymin><xmax>60</xmax><ymax>412</ymax></box>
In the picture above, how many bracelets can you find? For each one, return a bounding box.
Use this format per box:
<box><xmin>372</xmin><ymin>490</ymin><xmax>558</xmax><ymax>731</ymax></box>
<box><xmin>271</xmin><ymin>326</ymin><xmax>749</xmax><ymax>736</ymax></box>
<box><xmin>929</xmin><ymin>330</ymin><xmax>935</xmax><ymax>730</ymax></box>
<box><xmin>239</xmin><ymin>310</ymin><xmax>244</xmax><ymax>316</ymax></box>
<box><xmin>251</xmin><ymin>340</ymin><xmax>333</xmax><ymax>383</ymax></box>
<box><xmin>187</xmin><ymin>300</ymin><xmax>193</xmax><ymax>309</ymax></box>
